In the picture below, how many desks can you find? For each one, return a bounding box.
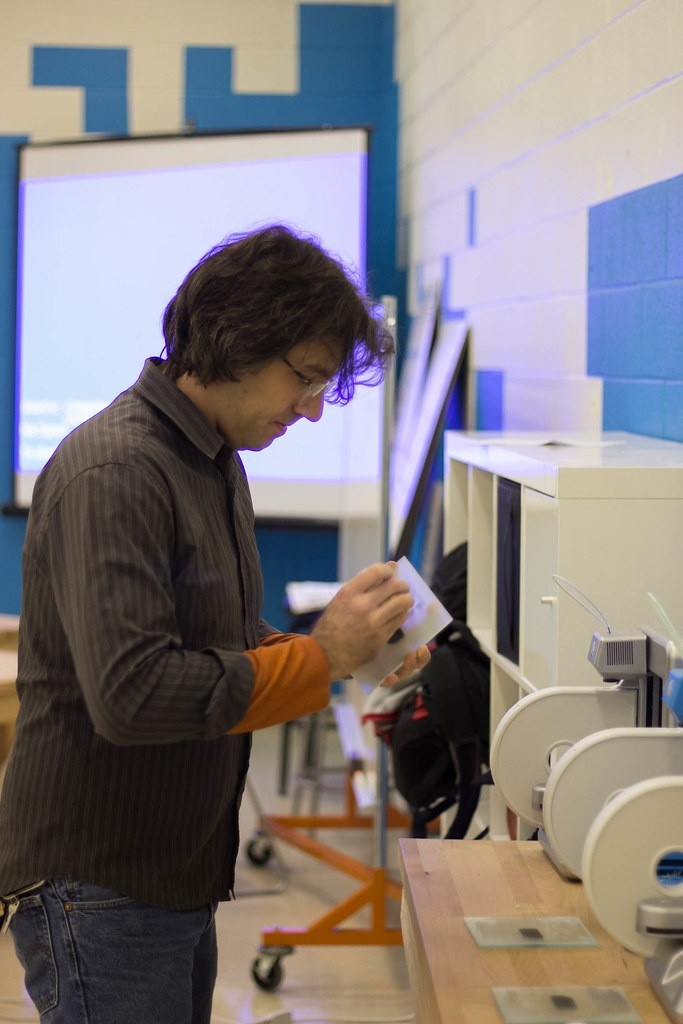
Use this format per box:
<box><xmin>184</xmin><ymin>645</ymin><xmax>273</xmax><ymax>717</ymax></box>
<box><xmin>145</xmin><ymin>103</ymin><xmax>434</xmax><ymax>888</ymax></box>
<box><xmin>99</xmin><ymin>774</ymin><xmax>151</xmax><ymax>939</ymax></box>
<box><xmin>275</xmin><ymin>580</ymin><xmax>354</xmax><ymax>799</ymax></box>
<box><xmin>395</xmin><ymin>838</ymin><xmax>683</xmax><ymax>1024</ymax></box>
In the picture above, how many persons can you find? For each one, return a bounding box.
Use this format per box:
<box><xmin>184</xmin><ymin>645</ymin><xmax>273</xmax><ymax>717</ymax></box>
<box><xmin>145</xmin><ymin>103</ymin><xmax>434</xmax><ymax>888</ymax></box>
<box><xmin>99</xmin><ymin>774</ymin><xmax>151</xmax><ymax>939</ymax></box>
<box><xmin>0</xmin><ymin>222</ymin><xmax>431</xmax><ymax>1024</ymax></box>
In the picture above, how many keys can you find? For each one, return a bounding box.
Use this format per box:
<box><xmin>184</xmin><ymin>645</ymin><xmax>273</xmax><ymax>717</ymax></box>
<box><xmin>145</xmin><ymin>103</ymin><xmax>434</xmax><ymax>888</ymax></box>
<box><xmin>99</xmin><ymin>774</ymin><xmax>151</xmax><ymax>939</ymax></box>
<box><xmin>3</xmin><ymin>899</ymin><xmax>19</xmax><ymax>934</ymax></box>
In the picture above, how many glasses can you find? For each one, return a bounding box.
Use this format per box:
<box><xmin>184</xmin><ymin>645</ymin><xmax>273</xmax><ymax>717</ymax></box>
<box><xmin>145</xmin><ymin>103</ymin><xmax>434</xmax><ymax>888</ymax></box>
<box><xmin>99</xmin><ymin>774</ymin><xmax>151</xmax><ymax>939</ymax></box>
<box><xmin>280</xmin><ymin>355</ymin><xmax>337</xmax><ymax>408</ymax></box>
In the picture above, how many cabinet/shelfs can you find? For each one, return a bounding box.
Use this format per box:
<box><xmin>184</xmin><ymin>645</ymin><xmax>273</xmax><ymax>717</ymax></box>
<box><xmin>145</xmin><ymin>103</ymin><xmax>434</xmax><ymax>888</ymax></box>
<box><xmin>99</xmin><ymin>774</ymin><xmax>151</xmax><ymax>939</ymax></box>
<box><xmin>442</xmin><ymin>422</ymin><xmax>683</xmax><ymax>846</ymax></box>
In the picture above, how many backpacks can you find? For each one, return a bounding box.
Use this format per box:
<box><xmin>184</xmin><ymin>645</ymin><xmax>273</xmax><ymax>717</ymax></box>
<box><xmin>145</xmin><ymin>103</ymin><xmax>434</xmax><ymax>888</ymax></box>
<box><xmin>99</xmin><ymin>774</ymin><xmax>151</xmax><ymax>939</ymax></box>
<box><xmin>394</xmin><ymin>624</ymin><xmax>489</xmax><ymax>841</ymax></box>
